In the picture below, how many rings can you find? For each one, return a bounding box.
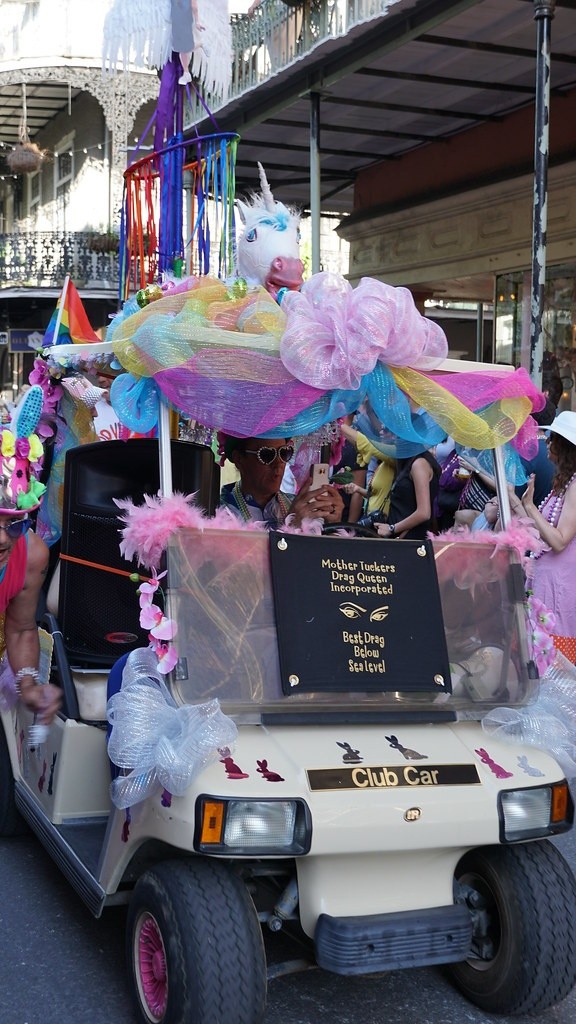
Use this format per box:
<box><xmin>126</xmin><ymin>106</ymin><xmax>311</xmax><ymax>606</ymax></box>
<box><xmin>330</xmin><ymin>505</ymin><xmax>336</xmax><ymax>514</ymax></box>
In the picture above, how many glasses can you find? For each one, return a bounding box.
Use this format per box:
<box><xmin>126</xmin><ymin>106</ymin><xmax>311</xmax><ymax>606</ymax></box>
<box><xmin>243</xmin><ymin>445</ymin><xmax>295</xmax><ymax>467</ymax></box>
<box><xmin>0</xmin><ymin>518</ymin><xmax>36</xmax><ymax>539</ymax></box>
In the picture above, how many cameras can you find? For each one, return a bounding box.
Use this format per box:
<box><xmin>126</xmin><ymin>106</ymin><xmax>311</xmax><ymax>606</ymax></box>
<box><xmin>356</xmin><ymin>510</ymin><xmax>385</xmax><ymax>534</ymax></box>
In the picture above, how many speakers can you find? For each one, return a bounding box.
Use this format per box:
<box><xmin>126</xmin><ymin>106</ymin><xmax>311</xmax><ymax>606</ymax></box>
<box><xmin>58</xmin><ymin>438</ymin><xmax>222</xmax><ymax>674</ymax></box>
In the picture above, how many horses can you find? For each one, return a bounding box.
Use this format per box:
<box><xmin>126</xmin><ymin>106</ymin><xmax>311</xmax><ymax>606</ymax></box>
<box><xmin>232</xmin><ymin>190</ymin><xmax>305</xmax><ymax>303</ymax></box>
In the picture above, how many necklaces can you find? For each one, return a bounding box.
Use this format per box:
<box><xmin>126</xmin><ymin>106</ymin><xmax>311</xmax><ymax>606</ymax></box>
<box><xmin>232</xmin><ymin>479</ymin><xmax>288</xmax><ymax>524</ymax></box>
<box><xmin>530</xmin><ymin>473</ymin><xmax>576</xmax><ymax>559</ymax></box>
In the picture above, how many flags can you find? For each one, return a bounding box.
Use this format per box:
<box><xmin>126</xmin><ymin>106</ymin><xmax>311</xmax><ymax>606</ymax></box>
<box><xmin>42</xmin><ymin>280</ymin><xmax>101</xmax><ymax>348</ymax></box>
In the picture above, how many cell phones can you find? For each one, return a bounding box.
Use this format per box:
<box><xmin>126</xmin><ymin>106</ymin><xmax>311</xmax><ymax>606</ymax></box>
<box><xmin>308</xmin><ymin>463</ymin><xmax>329</xmax><ymax>512</ymax></box>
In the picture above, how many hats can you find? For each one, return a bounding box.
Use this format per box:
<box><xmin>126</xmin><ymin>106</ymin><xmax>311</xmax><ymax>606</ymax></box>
<box><xmin>534</xmin><ymin>410</ymin><xmax>576</xmax><ymax>445</ymax></box>
<box><xmin>0</xmin><ymin>385</ymin><xmax>47</xmax><ymax>513</ymax></box>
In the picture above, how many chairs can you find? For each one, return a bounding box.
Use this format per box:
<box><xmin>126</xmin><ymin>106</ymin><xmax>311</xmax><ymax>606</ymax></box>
<box><xmin>51</xmin><ymin>434</ymin><xmax>223</xmax><ymax>740</ymax></box>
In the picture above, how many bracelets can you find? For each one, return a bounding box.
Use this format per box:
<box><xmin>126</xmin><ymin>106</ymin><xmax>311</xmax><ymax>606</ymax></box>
<box><xmin>475</xmin><ymin>468</ymin><xmax>481</xmax><ymax>473</ymax></box>
<box><xmin>14</xmin><ymin>667</ymin><xmax>39</xmax><ymax>697</ymax></box>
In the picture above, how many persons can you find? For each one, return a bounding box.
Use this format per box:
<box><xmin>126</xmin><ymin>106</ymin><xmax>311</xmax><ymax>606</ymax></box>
<box><xmin>217</xmin><ymin>430</ymin><xmax>344</xmax><ymax>536</ymax></box>
<box><xmin>62</xmin><ymin>377</ymin><xmax>109</xmax><ymax>438</ymax></box>
<box><xmin>94</xmin><ymin>376</ymin><xmax>134</xmax><ymax>441</ymax></box>
<box><xmin>0</xmin><ymin>512</ymin><xmax>62</xmax><ymax>726</ymax></box>
<box><xmin>280</xmin><ymin>370</ymin><xmax>576</xmax><ymax>665</ymax></box>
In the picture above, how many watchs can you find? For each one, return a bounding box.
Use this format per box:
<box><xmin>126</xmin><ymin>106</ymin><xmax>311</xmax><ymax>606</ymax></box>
<box><xmin>389</xmin><ymin>525</ymin><xmax>395</xmax><ymax>533</ymax></box>
